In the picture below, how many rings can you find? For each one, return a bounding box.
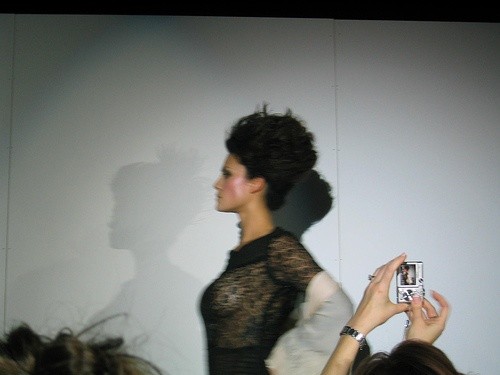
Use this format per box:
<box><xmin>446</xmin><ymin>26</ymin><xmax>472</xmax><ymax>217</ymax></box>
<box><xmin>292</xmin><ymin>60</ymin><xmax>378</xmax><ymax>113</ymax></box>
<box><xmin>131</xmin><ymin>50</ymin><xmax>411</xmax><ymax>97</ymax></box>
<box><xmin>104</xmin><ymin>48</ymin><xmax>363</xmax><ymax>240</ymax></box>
<box><xmin>368</xmin><ymin>275</ymin><xmax>375</xmax><ymax>280</ymax></box>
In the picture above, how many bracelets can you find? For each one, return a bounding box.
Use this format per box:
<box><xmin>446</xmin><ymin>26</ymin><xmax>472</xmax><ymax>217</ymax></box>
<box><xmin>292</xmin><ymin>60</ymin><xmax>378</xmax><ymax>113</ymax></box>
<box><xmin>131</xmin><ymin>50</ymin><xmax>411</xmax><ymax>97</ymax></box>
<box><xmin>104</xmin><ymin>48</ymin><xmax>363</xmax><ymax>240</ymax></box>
<box><xmin>340</xmin><ymin>326</ymin><xmax>368</xmax><ymax>351</ymax></box>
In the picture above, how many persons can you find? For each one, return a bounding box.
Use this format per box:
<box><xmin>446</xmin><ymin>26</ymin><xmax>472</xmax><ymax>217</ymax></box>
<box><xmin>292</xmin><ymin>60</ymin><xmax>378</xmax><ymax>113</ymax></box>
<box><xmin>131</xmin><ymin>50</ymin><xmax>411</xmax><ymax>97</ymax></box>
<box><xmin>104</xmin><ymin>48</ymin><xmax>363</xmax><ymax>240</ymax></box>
<box><xmin>199</xmin><ymin>111</ymin><xmax>353</xmax><ymax>375</ymax></box>
<box><xmin>322</xmin><ymin>253</ymin><xmax>465</xmax><ymax>375</ymax></box>
<box><xmin>401</xmin><ymin>265</ymin><xmax>412</xmax><ymax>285</ymax></box>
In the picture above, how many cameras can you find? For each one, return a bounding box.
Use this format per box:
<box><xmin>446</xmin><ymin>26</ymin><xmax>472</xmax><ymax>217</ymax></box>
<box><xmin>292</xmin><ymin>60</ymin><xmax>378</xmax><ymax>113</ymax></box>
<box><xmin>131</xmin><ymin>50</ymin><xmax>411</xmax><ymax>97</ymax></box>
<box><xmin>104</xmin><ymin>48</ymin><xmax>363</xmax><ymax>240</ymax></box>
<box><xmin>397</xmin><ymin>262</ymin><xmax>425</xmax><ymax>304</ymax></box>
